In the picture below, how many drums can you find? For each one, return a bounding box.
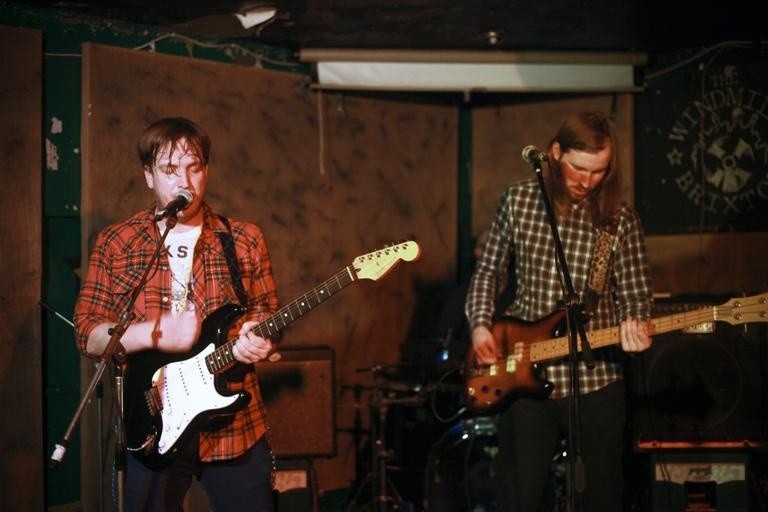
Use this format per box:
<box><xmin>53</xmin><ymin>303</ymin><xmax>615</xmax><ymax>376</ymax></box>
<box><xmin>425</xmin><ymin>416</ymin><xmax>567</xmax><ymax>512</ymax></box>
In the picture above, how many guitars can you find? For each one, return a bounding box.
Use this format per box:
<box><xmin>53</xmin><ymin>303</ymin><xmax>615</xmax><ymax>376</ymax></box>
<box><xmin>463</xmin><ymin>293</ymin><xmax>768</xmax><ymax>419</ymax></box>
<box><xmin>123</xmin><ymin>238</ymin><xmax>421</xmax><ymax>472</ymax></box>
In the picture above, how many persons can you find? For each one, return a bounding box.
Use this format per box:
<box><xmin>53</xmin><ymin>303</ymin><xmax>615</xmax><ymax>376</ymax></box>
<box><xmin>463</xmin><ymin>108</ymin><xmax>652</xmax><ymax>512</ymax></box>
<box><xmin>73</xmin><ymin>116</ymin><xmax>282</xmax><ymax>512</ymax></box>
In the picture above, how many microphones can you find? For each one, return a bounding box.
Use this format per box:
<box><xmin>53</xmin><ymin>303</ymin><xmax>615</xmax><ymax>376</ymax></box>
<box><xmin>151</xmin><ymin>190</ymin><xmax>195</xmax><ymax>222</ymax></box>
<box><xmin>521</xmin><ymin>143</ymin><xmax>548</xmax><ymax>166</ymax></box>
